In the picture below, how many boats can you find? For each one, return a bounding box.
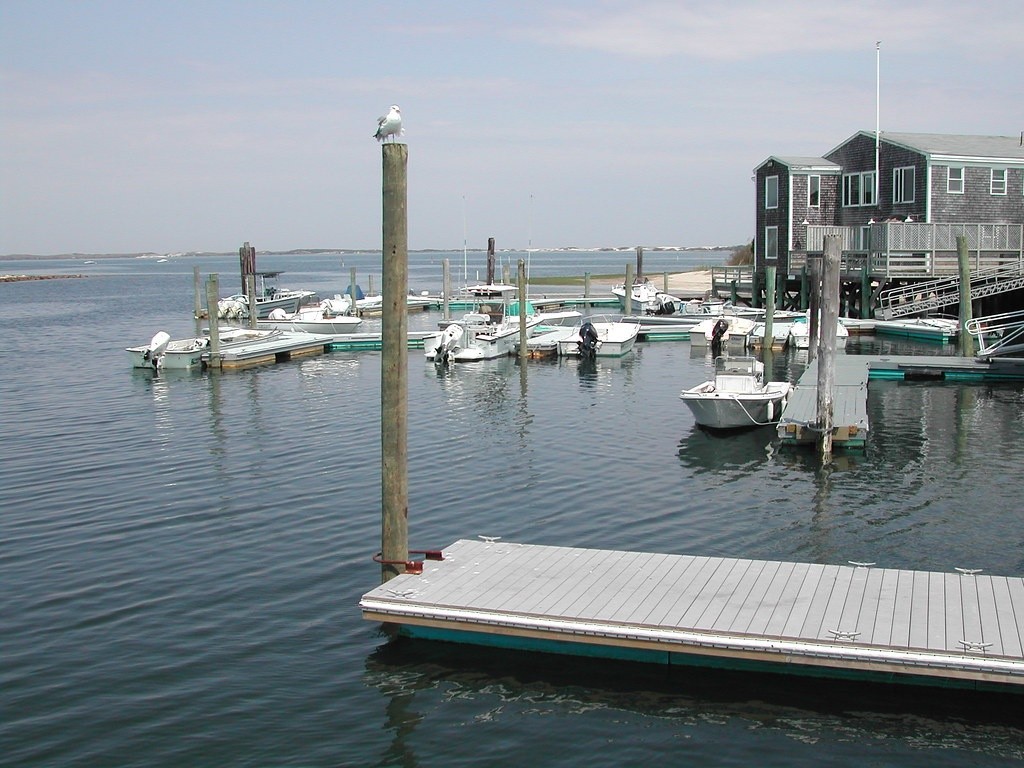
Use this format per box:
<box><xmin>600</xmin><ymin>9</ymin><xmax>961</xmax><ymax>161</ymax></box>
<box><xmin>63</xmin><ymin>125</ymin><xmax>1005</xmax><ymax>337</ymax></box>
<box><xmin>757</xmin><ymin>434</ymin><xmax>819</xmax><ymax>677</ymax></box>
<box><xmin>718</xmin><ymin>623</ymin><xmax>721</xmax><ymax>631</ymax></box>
<box><xmin>267</xmin><ymin>309</ymin><xmax>363</xmax><ymax>333</ymax></box>
<box><xmin>611</xmin><ymin>282</ymin><xmax>724</xmax><ymax>317</ymax></box>
<box><xmin>125</xmin><ymin>326</ymin><xmax>284</xmax><ymax>372</ymax></box>
<box><xmin>218</xmin><ymin>271</ymin><xmax>316</xmax><ymax>319</ymax></box>
<box><xmin>678</xmin><ymin>355</ymin><xmax>791</xmax><ymax>429</ymax></box>
<box><xmin>688</xmin><ymin>311</ymin><xmax>848</xmax><ymax>352</ymax></box>
<box><xmin>424</xmin><ymin>194</ymin><xmax>544</xmax><ymax>370</ymax></box>
<box><xmin>557</xmin><ymin>314</ymin><xmax>641</xmax><ymax>358</ymax></box>
<box><xmin>928</xmin><ymin>313</ymin><xmax>1004</xmax><ymax>339</ymax></box>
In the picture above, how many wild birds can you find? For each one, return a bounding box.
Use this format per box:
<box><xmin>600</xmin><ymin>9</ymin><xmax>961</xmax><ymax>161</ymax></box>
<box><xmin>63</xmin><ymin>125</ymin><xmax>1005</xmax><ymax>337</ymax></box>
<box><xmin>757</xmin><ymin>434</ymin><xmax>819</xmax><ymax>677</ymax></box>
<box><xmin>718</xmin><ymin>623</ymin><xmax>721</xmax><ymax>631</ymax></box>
<box><xmin>372</xmin><ymin>105</ymin><xmax>404</xmax><ymax>144</ymax></box>
